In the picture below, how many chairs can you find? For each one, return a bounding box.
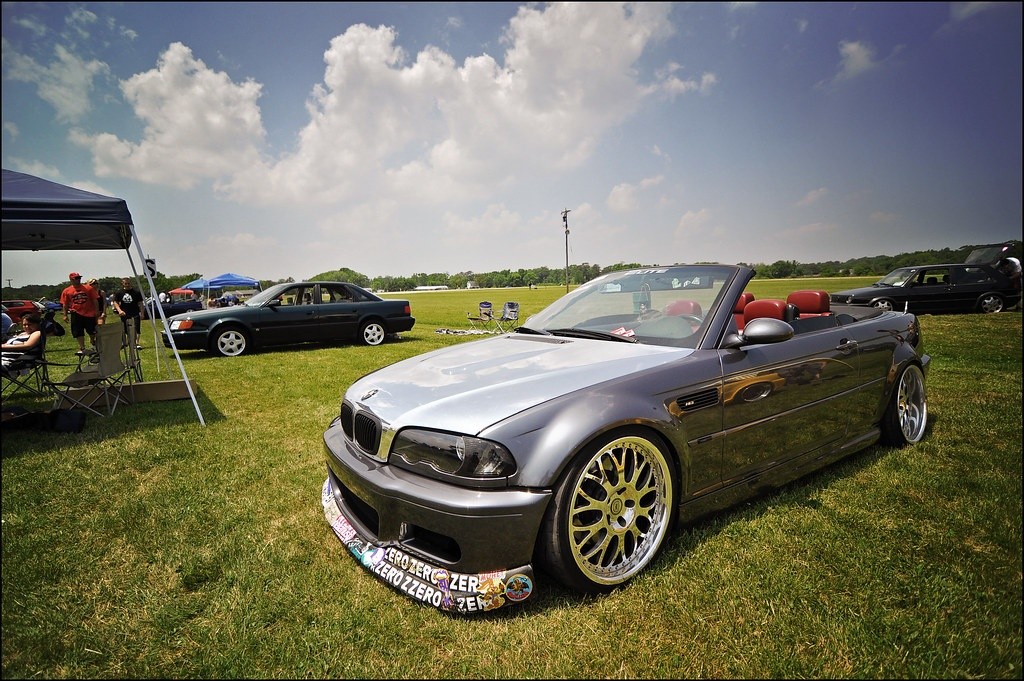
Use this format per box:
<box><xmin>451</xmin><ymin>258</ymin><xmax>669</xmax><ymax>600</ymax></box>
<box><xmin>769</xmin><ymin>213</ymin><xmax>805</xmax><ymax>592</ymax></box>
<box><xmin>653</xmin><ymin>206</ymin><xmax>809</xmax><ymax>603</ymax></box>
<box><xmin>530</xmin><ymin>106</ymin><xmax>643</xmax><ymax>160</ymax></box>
<box><xmin>927</xmin><ymin>277</ymin><xmax>937</xmax><ymax>283</ymax></box>
<box><xmin>489</xmin><ymin>302</ymin><xmax>520</xmax><ymax>334</ymax></box>
<box><xmin>302</xmin><ymin>292</ymin><xmax>311</xmax><ymax>305</ymax></box>
<box><xmin>670</xmin><ymin>300</ymin><xmax>701</xmax><ymax>326</ymax></box>
<box><xmin>744</xmin><ymin>299</ymin><xmax>800</xmax><ymax>325</ymax></box>
<box><xmin>467</xmin><ymin>301</ymin><xmax>494</xmax><ymax>331</ymax></box>
<box><xmin>786</xmin><ymin>290</ymin><xmax>832</xmax><ymax>319</ymax></box>
<box><xmin>1</xmin><ymin>313</ymin><xmax>143</xmax><ymax>417</ymax></box>
<box><xmin>733</xmin><ymin>292</ymin><xmax>754</xmax><ymax>329</ymax></box>
<box><xmin>942</xmin><ymin>275</ymin><xmax>949</xmax><ymax>283</ymax></box>
<box><xmin>287</xmin><ymin>298</ymin><xmax>294</xmax><ymax>306</ymax></box>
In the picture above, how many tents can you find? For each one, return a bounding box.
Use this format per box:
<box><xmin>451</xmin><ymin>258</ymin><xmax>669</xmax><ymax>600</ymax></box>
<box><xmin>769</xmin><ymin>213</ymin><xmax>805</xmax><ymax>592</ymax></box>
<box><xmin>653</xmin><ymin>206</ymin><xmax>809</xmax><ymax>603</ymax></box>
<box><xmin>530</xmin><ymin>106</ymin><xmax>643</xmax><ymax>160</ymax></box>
<box><xmin>168</xmin><ymin>272</ymin><xmax>263</xmax><ymax>303</ymax></box>
<box><xmin>0</xmin><ymin>169</ymin><xmax>208</xmax><ymax>428</ymax></box>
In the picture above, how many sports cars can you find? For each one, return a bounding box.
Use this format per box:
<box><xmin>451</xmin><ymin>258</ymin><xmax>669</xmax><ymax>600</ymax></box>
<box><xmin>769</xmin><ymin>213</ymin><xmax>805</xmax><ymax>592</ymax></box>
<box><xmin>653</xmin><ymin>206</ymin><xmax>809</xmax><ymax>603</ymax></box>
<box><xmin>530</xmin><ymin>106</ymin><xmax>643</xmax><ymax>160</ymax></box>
<box><xmin>321</xmin><ymin>264</ymin><xmax>930</xmax><ymax>616</ymax></box>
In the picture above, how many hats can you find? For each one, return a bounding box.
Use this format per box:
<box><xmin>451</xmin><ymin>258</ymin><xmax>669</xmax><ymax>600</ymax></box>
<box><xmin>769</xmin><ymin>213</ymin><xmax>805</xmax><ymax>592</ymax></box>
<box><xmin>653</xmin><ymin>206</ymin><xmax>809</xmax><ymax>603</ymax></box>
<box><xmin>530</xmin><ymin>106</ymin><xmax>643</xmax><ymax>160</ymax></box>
<box><xmin>69</xmin><ymin>272</ymin><xmax>83</xmax><ymax>280</ymax></box>
<box><xmin>88</xmin><ymin>279</ymin><xmax>97</xmax><ymax>285</ymax></box>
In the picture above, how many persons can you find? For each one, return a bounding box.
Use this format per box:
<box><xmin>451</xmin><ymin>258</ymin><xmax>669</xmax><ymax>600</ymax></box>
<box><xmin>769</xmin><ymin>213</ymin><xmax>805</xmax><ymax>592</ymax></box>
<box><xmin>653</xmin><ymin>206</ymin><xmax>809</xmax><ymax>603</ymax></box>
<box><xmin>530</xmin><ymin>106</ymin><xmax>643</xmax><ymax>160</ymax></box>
<box><xmin>159</xmin><ymin>291</ymin><xmax>166</xmax><ymax>303</ymax></box>
<box><xmin>87</xmin><ymin>278</ymin><xmax>107</xmax><ymax>325</ymax></box>
<box><xmin>207</xmin><ymin>294</ymin><xmax>217</xmax><ymax>310</ymax></box>
<box><xmin>996</xmin><ymin>257</ymin><xmax>1022</xmax><ymax>310</ymax></box>
<box><xmin>196</xmin><ymin>293</ymin><xmax>207</xmax><ymax>309</ymax></box>
<box><xmin>0</xmin><ymin>313</ymin><xmax>41</xmax><ymax>366</ymax></box>
<box><xmin>60</xmin><ymin>272</ymin><xmax>101</xmax><ymax>355</ymax></box>
<box><xmin>167</xmin><ymin>293</ymin><xmax>171</xmax><ymax>303</ymax></box>
<box><xmin>110</xmin><ymin>290</ymin><xmax>115</xmax><ymax>316</ymax></box>
<box><xmin>528</xmin><ymin>282</ymin><xmax>532</xmax><ymax>290</ymax></box>
<box><xmin>115</xmin><ymin>277</ymin><xmax>145</xmax><ymax>349</ymax></box>
<box><xmin>188</xmin><ymin>290</ymin><xmax>196</xmax><ymax>301</ymax></box>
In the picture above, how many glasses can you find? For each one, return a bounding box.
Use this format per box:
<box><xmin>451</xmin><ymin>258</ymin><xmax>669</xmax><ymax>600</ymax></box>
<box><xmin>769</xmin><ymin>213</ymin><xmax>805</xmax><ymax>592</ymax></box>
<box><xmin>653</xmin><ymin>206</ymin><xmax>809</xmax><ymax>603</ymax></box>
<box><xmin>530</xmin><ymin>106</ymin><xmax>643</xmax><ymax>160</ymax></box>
<box><xmin>73</xmin><ymin>277</ymin><xmax>81</xmax><ymax>280</ymax></box>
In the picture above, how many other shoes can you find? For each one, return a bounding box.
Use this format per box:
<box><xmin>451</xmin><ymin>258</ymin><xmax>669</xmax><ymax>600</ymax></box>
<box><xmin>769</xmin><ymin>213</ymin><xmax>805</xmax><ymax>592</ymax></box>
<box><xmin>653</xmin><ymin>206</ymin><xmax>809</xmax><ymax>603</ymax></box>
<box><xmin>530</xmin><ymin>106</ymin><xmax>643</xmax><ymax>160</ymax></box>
<box><xmin>90</xmin><ymin>340</ymin><xmax>96</xmax><ymax>346</ymax></box>
<box><xmin>137</xmin><ymin>346</ymin><xmax>142</xmax><ymax>348</ymax></box>
<box><xmin>77</xmin><ymin>348</ymin><xmax>85</xmax><ymax>354</ymax></box>
<box><xmin>121</xmin><ymin>344</ymin><xmax>128</xmax><ymax>349</ymax></box>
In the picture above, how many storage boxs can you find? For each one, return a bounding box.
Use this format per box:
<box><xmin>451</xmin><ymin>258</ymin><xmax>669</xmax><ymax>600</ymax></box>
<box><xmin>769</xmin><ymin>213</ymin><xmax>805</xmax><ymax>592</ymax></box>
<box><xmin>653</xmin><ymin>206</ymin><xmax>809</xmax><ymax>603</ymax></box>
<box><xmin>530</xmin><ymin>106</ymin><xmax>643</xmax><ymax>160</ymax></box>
<box><xmin>60</xmin><ymin>378</ymin><xmax>198</xmax><ymax>409</ymax></box>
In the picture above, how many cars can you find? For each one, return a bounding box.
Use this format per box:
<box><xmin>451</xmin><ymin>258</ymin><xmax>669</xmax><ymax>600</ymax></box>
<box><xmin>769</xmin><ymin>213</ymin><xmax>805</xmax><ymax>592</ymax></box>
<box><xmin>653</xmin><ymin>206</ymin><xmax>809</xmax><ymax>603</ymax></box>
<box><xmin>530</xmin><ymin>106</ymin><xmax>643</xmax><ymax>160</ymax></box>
<box><xmin>1</xmin><ymin>297</ymin><xmax>65</xmax><ymax>326</ymax></box>
<box><xmin>159</xmin><ymin>280</ymin><xmax>416</xmax><ymax>357</ymax></box>
<box><xmin>830</xmin><ymin>240</ymin><xmax>1022</xmax><ymax>313</ymax></box>
<box><xmin>138</xmin><ymin>294</ymin><xmax>205</xmax><ymax>320</ymax></box>
